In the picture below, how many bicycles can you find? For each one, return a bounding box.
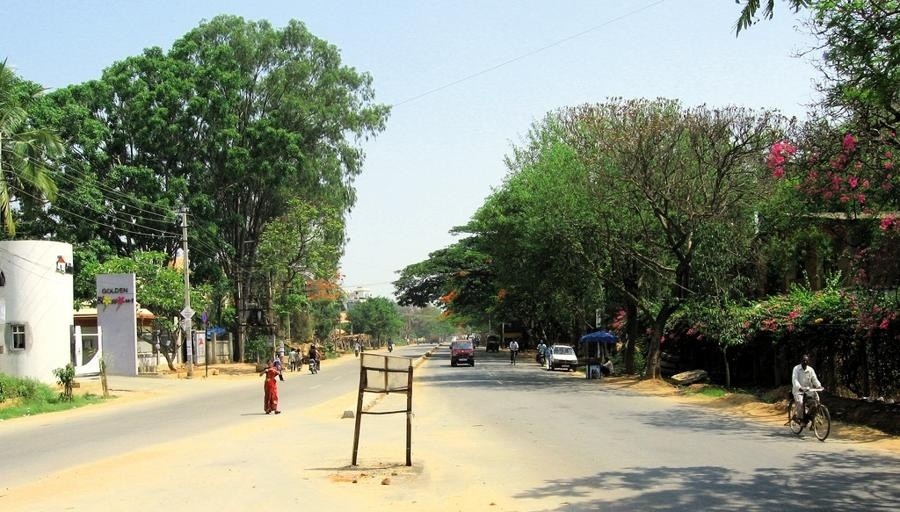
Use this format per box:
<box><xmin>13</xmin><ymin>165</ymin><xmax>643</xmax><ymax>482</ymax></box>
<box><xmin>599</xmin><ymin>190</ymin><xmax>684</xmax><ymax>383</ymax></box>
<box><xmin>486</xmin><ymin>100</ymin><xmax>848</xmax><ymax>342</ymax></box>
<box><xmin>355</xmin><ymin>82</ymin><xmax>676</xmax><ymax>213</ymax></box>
<box><xmin>788</xmin><ymin>385</ymin><xmax>833</xmax><ymax>442</ymax></box>
<box><xmin>510</xmin><ymin>349</ymin><xmax>520</xmax><ymax>367</ymax></box>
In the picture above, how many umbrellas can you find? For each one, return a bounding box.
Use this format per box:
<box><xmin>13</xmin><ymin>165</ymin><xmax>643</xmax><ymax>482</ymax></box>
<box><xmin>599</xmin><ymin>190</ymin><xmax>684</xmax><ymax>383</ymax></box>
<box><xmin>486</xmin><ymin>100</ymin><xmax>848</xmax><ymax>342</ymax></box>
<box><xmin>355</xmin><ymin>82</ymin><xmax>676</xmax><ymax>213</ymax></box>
<box><xmin>578</xmin><ymin>331</ymin><xmax>618</xmax><ymax>358</ymax></box>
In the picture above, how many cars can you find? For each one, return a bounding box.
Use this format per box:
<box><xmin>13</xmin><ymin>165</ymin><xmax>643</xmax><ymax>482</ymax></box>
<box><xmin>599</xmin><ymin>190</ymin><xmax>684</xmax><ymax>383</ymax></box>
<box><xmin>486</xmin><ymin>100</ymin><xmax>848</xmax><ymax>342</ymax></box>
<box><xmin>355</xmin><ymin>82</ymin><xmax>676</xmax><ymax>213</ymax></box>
<box><xmin>547</xmin><ymin>344</ymin><xmax>581</xmax><ymax>373</ymax></box>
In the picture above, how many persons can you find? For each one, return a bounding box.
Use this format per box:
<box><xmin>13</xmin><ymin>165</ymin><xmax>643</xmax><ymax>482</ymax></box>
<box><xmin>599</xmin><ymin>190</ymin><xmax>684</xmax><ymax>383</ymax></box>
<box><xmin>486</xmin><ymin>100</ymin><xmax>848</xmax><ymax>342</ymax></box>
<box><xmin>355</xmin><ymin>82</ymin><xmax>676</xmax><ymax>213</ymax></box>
<box><xmin>353</xmin><ymin>342</ymin><xmax>361</xmax><ymax>355</ymax></box>
<box><xmin>295</xmin><ymin>349</ymin><xmax>302</xmax><ymax>371</ymax></box>
<box><xmin>790</xmin><ymin>354</ymin><xmax>824</xmax><ymax>430</ymax></box>
<box><xmin>601</xmin><ymin>357</ymin><xmax>615</xmax><ymax>376</ymax></box>
<box><xmin>272</xmin><ymin>358</ymin><xmax>285</xmax><ymax>381</ymax></box>
<box><xmin>536</xmin><ymin>339</ymin><xmax>548</xmax><ymax>363</ymax></box>
<box><xmin>509</xmin><ymin>339</ymin><xmax>519</xmax><ymax>363</ymax></box>
<box><xmin>388</xmin><ymin>338</ymin><xmax>393</xmax><ymax>350</ymax></box>
<box><xmin>259</xmin><ymin>359</ymin><xmax>282</xmax><ymax>414</ymax></box>
<box><xmin>307</xmin><ymin>344</ymin><xmax>321</xmax><ymax>370</ymax></box>
<box><xmin>288</xmin><ymin>348</ymin><xmax>296</xmax><ymax>372</ymax></box>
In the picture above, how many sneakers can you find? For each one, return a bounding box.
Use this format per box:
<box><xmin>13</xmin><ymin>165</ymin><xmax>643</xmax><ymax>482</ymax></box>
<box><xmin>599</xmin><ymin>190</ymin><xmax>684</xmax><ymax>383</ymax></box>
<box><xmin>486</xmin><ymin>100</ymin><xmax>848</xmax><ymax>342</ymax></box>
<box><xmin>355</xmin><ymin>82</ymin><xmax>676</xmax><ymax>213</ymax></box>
<box><xmin>265</xmin><ymin>410</ymin><xmax>281</xmax><ymax>415</ymax></box>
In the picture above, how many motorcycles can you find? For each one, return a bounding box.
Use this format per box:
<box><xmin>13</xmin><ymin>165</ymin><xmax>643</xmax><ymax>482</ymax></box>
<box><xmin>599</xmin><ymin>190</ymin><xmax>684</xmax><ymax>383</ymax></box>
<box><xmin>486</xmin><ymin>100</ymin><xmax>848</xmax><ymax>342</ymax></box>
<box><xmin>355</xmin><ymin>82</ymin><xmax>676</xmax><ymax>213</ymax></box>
<box><xmin>309</xmin><ymin>355</ymin><xmax>320</xmax><ymax>374</ymax></box>
<box><xmin>536</xmin><ymin>347</ymin><xmax>546</xmax><ymax>367</ymax></box>
<box><xmin>388</xmin><ymin>343</ymin><xmax>393</xmax><ymax>352</ymax></box>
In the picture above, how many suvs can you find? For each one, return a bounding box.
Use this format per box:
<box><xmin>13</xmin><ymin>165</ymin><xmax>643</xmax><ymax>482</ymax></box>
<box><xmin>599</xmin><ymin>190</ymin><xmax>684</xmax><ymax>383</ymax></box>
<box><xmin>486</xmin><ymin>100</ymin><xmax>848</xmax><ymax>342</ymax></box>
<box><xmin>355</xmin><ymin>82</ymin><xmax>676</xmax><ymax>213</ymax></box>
<box><xmin>448</xmin><ymin>335</ymin><xmax>501</xmax><ymax>367</ymax></box>
